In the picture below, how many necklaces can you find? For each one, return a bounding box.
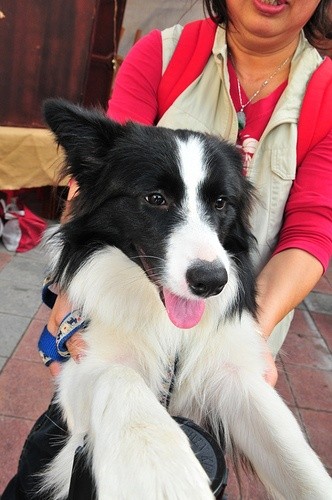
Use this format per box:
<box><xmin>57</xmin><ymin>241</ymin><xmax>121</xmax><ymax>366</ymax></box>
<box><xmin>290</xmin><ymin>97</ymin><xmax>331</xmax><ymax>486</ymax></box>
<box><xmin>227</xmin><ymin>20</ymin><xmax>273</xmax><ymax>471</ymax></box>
<box><xmin>223</xmin><ymin>37</ymin><xmax>294</xmax><ymax>136</ymax></box>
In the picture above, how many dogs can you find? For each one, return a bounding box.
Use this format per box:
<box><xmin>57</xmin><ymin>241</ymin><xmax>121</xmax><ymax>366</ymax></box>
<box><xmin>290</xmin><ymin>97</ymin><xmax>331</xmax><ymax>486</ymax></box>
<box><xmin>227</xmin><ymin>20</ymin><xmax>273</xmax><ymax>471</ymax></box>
<box><xmin>0</xmin><ymin>96</ymin><xmax>332</xmax><ymax>500</ymax></box>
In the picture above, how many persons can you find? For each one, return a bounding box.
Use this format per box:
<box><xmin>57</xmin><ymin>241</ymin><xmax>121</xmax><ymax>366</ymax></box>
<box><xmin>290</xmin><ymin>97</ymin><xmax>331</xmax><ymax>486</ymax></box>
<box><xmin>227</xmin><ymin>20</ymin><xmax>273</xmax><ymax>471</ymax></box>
<box><xmin>38</xmin><ymin>0</ymin><xmax>331</xmax><ymax>392</ymax></box>
